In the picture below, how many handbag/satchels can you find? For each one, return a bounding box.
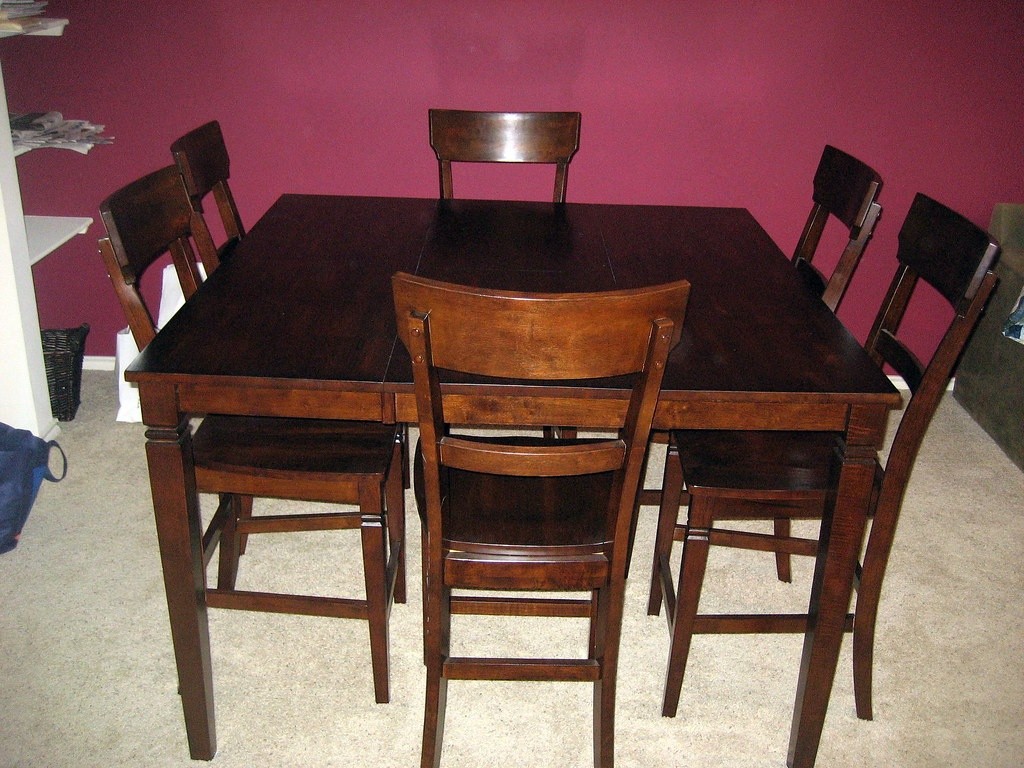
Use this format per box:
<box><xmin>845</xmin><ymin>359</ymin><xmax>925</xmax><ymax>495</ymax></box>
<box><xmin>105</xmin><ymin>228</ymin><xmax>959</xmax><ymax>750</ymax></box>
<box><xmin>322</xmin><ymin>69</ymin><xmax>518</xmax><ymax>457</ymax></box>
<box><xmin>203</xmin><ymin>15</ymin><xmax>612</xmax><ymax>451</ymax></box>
<box><xmin>0</xmin><ymin>422</ymin><xmax>68</xmax><ymax>554</ymax></box>
<box><xmin>116</xmin><ymin>322</ymin><xmax>142</xmax><ymax>423</ymax></box>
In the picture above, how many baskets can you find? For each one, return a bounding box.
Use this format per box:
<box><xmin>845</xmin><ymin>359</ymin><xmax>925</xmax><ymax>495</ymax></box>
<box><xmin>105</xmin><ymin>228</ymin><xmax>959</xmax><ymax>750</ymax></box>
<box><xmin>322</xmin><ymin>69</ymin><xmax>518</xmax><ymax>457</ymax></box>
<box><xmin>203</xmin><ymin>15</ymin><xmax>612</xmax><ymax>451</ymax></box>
<box><xmin>40</xmin><ymin>322</ymin><xmax>90</xmax><ymax>422</ymax></box>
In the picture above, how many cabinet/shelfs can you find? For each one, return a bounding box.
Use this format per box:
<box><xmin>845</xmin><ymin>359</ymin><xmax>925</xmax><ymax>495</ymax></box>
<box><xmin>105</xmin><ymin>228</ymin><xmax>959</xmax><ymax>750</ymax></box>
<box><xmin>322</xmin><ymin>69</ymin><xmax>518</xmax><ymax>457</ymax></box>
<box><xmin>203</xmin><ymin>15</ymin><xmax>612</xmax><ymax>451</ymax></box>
<box><xmin>0</xmin><ymin>19</ymin><xmax>93</xmax><ymax>269</ymax></box>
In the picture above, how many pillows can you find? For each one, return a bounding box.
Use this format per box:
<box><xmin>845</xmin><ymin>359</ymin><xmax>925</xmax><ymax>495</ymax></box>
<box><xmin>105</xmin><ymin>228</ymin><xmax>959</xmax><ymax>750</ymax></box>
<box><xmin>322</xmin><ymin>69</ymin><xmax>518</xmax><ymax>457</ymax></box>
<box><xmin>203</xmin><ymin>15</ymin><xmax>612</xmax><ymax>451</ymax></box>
<box><xmin>1002</xmin><ymin>286</ymin><xmax>1024</xmax><ymax>345</ymax></box>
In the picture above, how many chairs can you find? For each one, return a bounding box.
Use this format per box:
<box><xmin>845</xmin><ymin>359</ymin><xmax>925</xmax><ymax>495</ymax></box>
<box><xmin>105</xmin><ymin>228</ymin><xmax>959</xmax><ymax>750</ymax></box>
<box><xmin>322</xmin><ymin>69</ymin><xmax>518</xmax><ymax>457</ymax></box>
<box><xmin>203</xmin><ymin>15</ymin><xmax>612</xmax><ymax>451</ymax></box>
<box><xmin>644</xmin><ymin>194</ymin><xmax>996</xmax><ymax>719</ymax></box>
<box><xmin>388</xmin><ymin>269</ymin><xmax>692</xmax><ymax>768</ymax></box>
<box><xmin>640</xmin><ymin>143</ymin><xmax>882</xmax><ymax>583</ymax></box>
<box><xmin>97</xmin><ymin>164</ymin><xmax>406</xmax><ymax>703</ymax></box>
<box><xmin>170</xmin><ymin>120</ymin><xmax>245</xmax><ymax>276</ymax></box>
<box><xmin>427</xmin><ymin>104</ymin><xmax>581</xmax><ymax>201</ymax></box>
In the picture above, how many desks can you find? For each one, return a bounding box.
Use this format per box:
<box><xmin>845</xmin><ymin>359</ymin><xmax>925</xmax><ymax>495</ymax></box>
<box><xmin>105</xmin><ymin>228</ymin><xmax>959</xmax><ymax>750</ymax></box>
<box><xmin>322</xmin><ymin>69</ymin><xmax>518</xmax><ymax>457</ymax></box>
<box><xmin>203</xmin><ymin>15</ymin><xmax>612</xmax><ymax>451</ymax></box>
<box><xmin>125</xmin><ymin>194</ymin><xmax>904</xmax><ymax>768</ymax></box>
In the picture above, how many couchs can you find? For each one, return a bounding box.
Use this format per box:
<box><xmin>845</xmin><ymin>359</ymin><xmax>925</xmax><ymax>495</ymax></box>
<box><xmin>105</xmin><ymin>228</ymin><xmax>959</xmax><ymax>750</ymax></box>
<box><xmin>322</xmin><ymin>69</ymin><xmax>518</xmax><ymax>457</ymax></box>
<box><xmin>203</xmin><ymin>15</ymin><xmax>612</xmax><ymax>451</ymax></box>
<box><xmin>951</xmin><ymin>204</ymin><xmax>1024</xmax><ymax>473</ymax></box>
